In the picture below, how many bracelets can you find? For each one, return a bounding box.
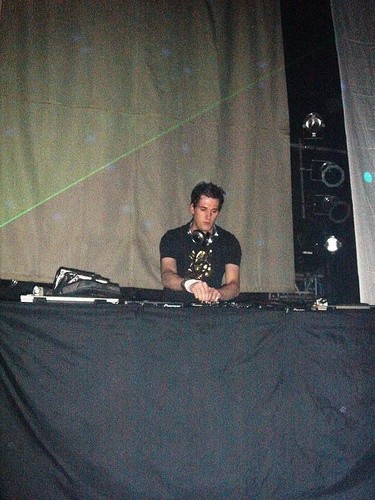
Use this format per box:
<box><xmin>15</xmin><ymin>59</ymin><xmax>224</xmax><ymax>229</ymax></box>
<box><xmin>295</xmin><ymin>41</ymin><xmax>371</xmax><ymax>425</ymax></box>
<box><xmin>181</xmin><ymin>277</ymin><xmax>201</xmax><ymax>293</ymax></box>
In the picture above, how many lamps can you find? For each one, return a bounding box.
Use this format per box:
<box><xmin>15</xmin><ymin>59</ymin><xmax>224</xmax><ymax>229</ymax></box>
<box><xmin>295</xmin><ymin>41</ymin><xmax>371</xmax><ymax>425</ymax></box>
<box><xmin>319</xmin><ymin>162</ymin><xmax>346</xmax><ymax>188</ymax></box>
<box><xmin>322</xmin><ymin>196</ymin><xmax>351</xmax><ymax>223</ymax></box>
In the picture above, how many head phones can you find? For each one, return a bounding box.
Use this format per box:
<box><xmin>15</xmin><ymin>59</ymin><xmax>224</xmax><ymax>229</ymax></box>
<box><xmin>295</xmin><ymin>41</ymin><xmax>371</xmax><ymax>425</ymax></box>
<box><xmin>188</xmin><ymin>221</ymin><xmax>219</xmax><ymax>246</ymax></box>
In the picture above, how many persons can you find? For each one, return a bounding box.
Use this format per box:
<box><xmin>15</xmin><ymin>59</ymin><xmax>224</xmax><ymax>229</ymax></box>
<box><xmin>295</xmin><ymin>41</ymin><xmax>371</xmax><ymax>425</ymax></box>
<box><xmin>159</xmin><ymin>182</ymin><xmax>242</xmax><ymax>304</ymax></box>
